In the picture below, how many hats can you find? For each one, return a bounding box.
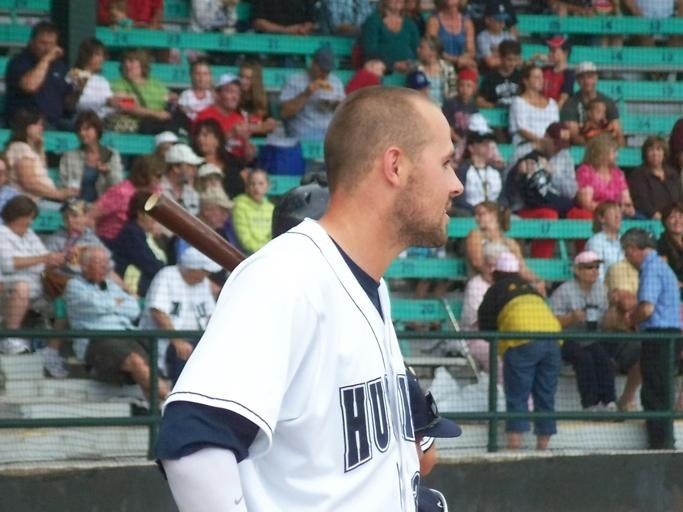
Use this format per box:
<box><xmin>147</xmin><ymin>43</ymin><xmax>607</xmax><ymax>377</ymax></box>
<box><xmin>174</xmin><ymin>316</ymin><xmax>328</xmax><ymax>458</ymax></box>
<box><xmin>547</xmin><ymin>36</ymin><xmax>571</xmax><ymax>54</ymax></box>
<box><xmin>315</xmin><ymin>47</ymin><xmax>338</xmax><ymax>72</ymax></box>
<box><xmin>59</xmin><ymin>198</ymin><xmax>86</xmax><ymax>213</ymax></box>
<box><xmin>494</xmin><ymin>252</ymin><xmax>518</xmax><ymax>273</ymax></box>
<box><xmin>214</xmin><ymin>74</ymin><xmax>241</xmax><ymax>88</ymax></box>
<box><xmin>180</xmin><ymin>245</ymin><xmax>224</xmax><ymax>274</ymax></box>
<box><xmin>155</xmin><ymin>132</ymin><xmax>179</xmax><ymax>146</ymax></box>
<box><xmin>548</xmin><ymin>123</ymin><xmax>573</xmax><ymax>148</ymax></box>
<box><xmin>486</xmin><ymin>3</ymin><xmax>510</xmax><ymax>20</ymax></box>
<box><xmin>575</xmin><ymin>62</ymin><xmax>598</xmax><ymax>76</ymax></box>
<box><xmin>459</xmin><ymin>69</ymin><xmax>478</xmax><ymax>84</ymax></box>
<box><xmin>574</xmin><ymin>252</ymin><xmax>603</xmax><ymax>264</ymax></box>
<box><xmin>197</xmin><ymin>163</ymin><xmax>221</xmax><ymax>178</ymax></box>
<box><xmin>406</xmin><ymin>367</ymin><xmax>461</xmax><ymax>438</ymax></box>
<box><xmin>468</xmin><ymin>130</ymin><xmax>495</xmax><ymax>142</ymax></box>
<box><xmin>166</xmin><ymin>144</ymin><xmax>204</xmax><ymax>165</ymax></box>
<box><xmin>199</xmin><ymin>189</ymin><xmax>233</xmax><ymax>208</ymax></box>
<box><xmin>408</xmin><ymin>72</ymin><xmax>430</xmax><ymax>90</ymax></box>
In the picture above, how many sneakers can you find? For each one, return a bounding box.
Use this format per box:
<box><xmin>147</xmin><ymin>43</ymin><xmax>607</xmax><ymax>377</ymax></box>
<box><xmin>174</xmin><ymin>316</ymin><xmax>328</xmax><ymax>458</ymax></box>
<box><xmin>39</xmin><ymin>348</ymin><xmax>68</xmax><ymax>378</ymax></box>
<box><xmin>1</xmin><ymin>337</ymin><xmax>29</xmax><ymax>354</ymax></box>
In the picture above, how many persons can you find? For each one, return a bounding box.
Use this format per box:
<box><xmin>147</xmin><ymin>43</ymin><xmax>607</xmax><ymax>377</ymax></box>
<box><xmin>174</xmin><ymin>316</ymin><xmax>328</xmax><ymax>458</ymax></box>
<box><xmin>0</xmin><ymin>196</ymin><xmax>70</xmax><ymax>380</ymax></box>
<box><xmin>279</xmin><ymin>46</ymin><xmax>347</xmax><ymax>141</ymax></box>
<box><xmin>110</xmin><ymin>191</ymin><xmax>169</xmax><ymax>292</ymax></box>
<box><xmin>65</xmin><ymin>36</ymin><xmax>115</xmax><ymax>128</ymax></box>
<box><xmin>93</xmin><ymin>154</ymin><xmax>164</xmax><ymax>243</ymax></box>
<box><xmin>321</xmin><ymin>1</ymin><xmax>375</xmax><ymax>35</ymax></box>
<box><xmin>631</xmin><ymin>135</ymin><xmax>683</xmax><ymax>218</ymax></box>
<box><xmin>605</xmin><ymin>256</ymin><xmax>642</xmax><ymax>413</ymax></box>
<box><xmin>426</xmin><ymin>0</ymin><xmax>476</xmax><ymax>69</ymax></box>
<box><xmin>64</xmin><ymin>245</ymin><xmax>170</xmax><ymax>402</ymax></box>
<box><xmin>107</xmin><ymin>46</ymin><xmax>178</xmax><ymax>134</ymax></box>
<box><xmin>6</xmin><ymin>24</ymin><xmax>69</xmax><ymax>130</ymax></box>
<box><xmin>230</xmin><ymin>168</ymin><xmax>275</xmax><ymax>254</ymax></box>
<box><xmin>406</xmin><ymin>33</ymin><xmax>457</xmax><ymax>106</ymax></box>
<box><xmin>576</xmin><ymin>132</ymin><xmax>637</xmax><ymax>218</ymax></box>
<box><xmin>138</xmin><ymin>245</ymin><xmax>224</xmax><ymax>388</ymax></box>
<box><xmin>408</xmin><ymin>71</ymin><xmax>431</xmax><ymax>94</ymax></box>
<box><xmin>561</xmin><ymin>59</ymin><xmax>622</xmax><ymax>144</ymax></box>
<box><xmin>461</xmin><ymin>240</ymin><xmax>519</xmax><ymax>387</ymax></box>
<box><xmin>358</xmin><ymin>0</ymin><xmax>423</xmax><ymax>86</ymax></box>
<box><xmin>59</xmin><ymin>109</ymin><xmax>123</xmax><ymax>205</ymax></box>
<box><xmin>502</xmin><ymin>124</ymin><xmax>593</xmax><ymax>258</ymax></box>
<box><xmin>238</xmin><ymin>60</ymin><xmax>275</xmax><ymax>139</ymax></box>
<box><xmin>584</xmin><ymin>98</ymin><xmax>611</xmax><ymax>135</ymax></box>
<box><xmin>466</xmin><ymin>203</ymin><xmax>535</xmax><ymax>286</ymax></box>
<box><xmin>454</xmin><ymin>133</ymin><xmax>520</xmax><ymax>222</ymax></box>
<box><xmin>176</xmin><ymin>53</ymin><xmax>216</xmax><ymax>132</ymax></box>
<box><xmin>186</xmin><ymin>0</ymin><xmax>239</xmax><ymax>33</ymax></box>
<box><xmin>197</xmin><ymin>160</ymin><xmax>224</xmax><ymax>189</ymax></box>
<box><xmin>95</xmin><ymin>1</ymin><xmax>165</xmax><ymax>30</ymax></box>
<box><xmin>530</xmin><ymin>35</ymin><xmax>575</xmax><ymax>104</ymax></box>
<box><xmin>197</xmin><ymin>74</ymin><xmax>256</xmax><ymax>166</ymax></box>
<box><xmin>550</xmin><ymin>250</ymin><xmax>617</xmax><ymax>411</ymax></box>
<box><xmin>174</xmin><ymin>186</ymin><xmax>231</xmax><ymax>265</ymax></box>
<box><xmin>474</xmin><ymin>40</ymin><xmax>529</xmax><ymax>107</ymax></box>
<box><xmin>249</xmin><ymin>0</ymin><xmax>319</xmax><ymax>34</ymax></box>
<box><xmin>584</xmin><ymin>199</ymin><xmax>627</xmax><ymax>283</ymax></box>
<box><xmin>142</xmin><ymin>87</ymin><xmax>451</xmax><ymax>512</ymax></box>
<box><xmin>479</xmin><ymin>252</ymin><xmax>563</xmax><ymax>448</ymax></box>
<box><xmin>445</xmin><ymin>69</ymin><xmax>497</xmax><ymax>146</ymax></box>
<box><xmin>620</xmin><ymin>229</ymin><xmax>682</xmax><ymax>451</ymax></box>
<box><xmin>5</xmin><ymin>108</ymin><xmax>73</xmax><ymax>210</ymax></box>
<box><xmin>270</xmin><ymin>173</ymin><xmax>333</xmax><ymax>239</ymax></box>
<box><xmin>162</xmin><ymin>141</ymin><xmax>205</xmax><ymax>218</ymax></box>
<box><xmin>475</xmin><ymin>3</ymin><xmax>517</xmax><ymax>69</ymax></box>
<box><xmin>509</xmin><ymin>65</ymin><xmax>562</xmax><ymax>158</ymax></box>
<box><xmin>657</xmin><ymin>200</ymin><xmax>682</xmax><ymax>299</ymax></box>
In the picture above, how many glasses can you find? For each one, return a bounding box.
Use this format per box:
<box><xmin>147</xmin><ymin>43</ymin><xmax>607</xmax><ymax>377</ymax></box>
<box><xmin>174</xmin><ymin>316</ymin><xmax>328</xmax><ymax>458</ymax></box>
<box><xmin>582</xmin><ymin>263</ymin><xmax>600</xmax><ymax>271</ymax></box>
